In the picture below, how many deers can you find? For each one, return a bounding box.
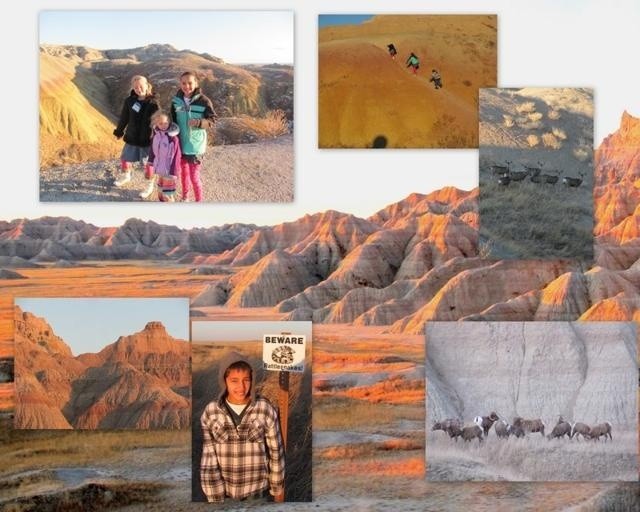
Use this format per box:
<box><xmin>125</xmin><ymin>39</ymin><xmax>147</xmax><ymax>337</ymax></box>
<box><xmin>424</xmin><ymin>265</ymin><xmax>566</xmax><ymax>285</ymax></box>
<box><xmin>490</xmin><ymin>160</ymin><xmax>586</xmax><ymax>191</ymax></box>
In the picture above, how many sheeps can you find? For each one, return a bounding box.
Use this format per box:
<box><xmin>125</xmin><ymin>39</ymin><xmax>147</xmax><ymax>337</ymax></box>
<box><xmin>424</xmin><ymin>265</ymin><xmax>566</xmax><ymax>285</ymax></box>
<box><xmin>431</xmin><ymin>411</ymin><xmax>612</xmax><ymax>443</ymax></box>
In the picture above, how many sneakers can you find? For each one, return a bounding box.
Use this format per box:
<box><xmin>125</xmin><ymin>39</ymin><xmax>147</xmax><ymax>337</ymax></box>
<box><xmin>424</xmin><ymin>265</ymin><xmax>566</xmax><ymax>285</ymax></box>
<box><xmin>138</xmin><ymin>177</ymin><xmax>156</xmax><ymax>199</ymax></box>
<box><xmin>112</xmin><ymin>170</ymin><xmax>132</xmax><ymax>187</ymax></box>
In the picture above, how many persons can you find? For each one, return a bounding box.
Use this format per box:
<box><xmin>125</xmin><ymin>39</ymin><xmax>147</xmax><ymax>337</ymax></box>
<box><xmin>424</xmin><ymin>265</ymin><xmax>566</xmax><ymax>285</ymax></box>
<box><xmin>113</xmin><ymin>72</ymin><xmax>217</xmax><ymax>202</ymax></box>
<box><xmin>405</xmin><ymin>52</ymin><xmax>419</xmax><ymax>74</ymax></box>
<box><xmin>388</xmin><ymin>44</ymin><xmax>397</xmax><ymax>60</ymax></box>
<box><xmin>430</xmin><ymin>69</ymin><xmax>442</xmax><ymax>89</ymax></box>
<box><xmin>201</xmin><ymin>352</ymin><xmax>286</xmax><ymax>504</ymax></box>
